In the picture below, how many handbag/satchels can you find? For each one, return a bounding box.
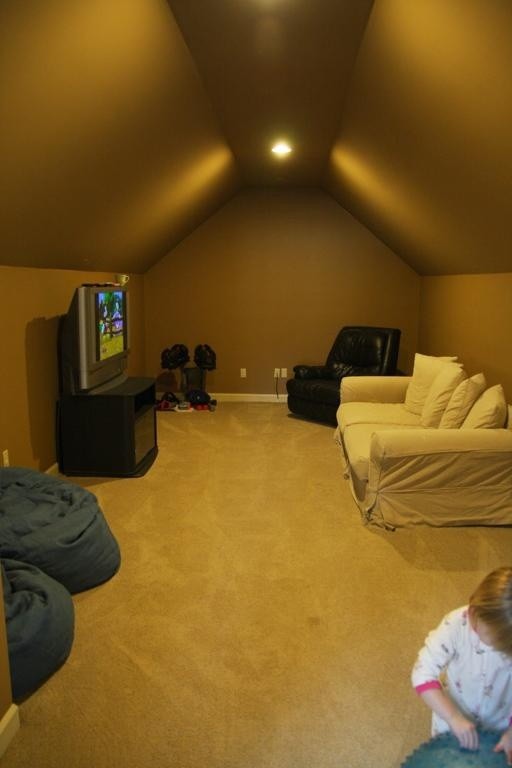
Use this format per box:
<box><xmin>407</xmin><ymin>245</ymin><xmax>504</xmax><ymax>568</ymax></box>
<box><xmin>194</xmin><ymin>342</ymin><xmax>216</xmax><ymax>371</ymax></box>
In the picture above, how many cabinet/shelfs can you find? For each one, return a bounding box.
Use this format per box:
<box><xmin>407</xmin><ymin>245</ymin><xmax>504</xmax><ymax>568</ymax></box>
<box><xmin>58</xmin><ymin>376</ymin><xmax>158</xmax><ymax>478</ymax></box>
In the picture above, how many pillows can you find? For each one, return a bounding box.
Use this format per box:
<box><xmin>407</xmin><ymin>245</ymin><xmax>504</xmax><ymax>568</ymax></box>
<box><xmin>404</xmin><ymin>352</ymin><xmax>508</xmax><ymax>430</ymax></box>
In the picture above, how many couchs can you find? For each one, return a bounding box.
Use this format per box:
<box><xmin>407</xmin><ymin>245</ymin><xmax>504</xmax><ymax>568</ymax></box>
<box><xmin>286</xmin><ymin>326</ymin><xmax>401</xmax><ymax>427</ymax></box>
<box><xmin>336</xmin><ymin>375</ymin><xmax>512</xmax><ymax>527</ymax></box>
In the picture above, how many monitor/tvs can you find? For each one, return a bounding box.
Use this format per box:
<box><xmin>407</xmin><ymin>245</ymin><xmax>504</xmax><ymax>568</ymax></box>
<box><xmin>62</xmin><ymin>284</ymin><xmax>131</xmax><ymax>395</ymax></box>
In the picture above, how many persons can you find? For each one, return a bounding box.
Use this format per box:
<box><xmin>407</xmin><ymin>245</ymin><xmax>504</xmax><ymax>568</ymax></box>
<box><xmin>410</xmin><ymin>567</ymin><xmax>512</xmax><ymax>766</ymax></box>
